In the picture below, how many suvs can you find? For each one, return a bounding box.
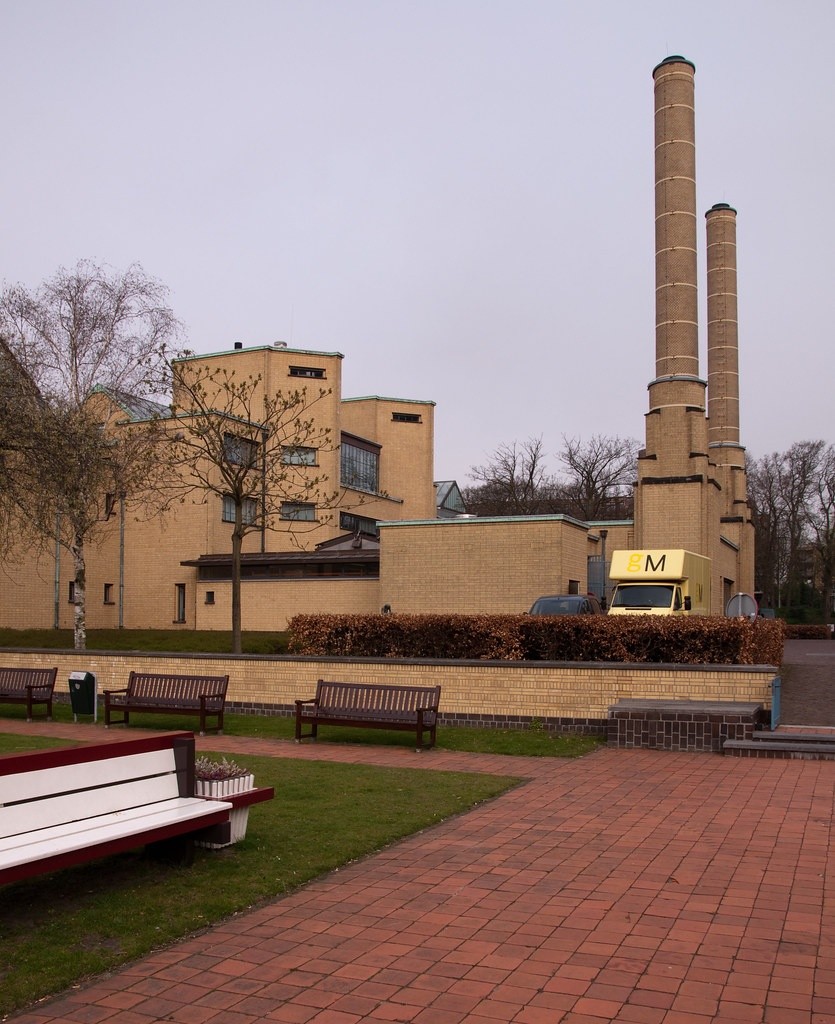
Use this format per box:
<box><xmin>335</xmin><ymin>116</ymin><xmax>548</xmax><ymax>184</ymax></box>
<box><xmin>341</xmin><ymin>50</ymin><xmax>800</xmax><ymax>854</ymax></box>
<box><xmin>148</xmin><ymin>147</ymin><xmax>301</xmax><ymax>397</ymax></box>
<box><xmin>526</xmin><ymin>592</ymin><xmax>602</xmax><ymax>619</ymax></box>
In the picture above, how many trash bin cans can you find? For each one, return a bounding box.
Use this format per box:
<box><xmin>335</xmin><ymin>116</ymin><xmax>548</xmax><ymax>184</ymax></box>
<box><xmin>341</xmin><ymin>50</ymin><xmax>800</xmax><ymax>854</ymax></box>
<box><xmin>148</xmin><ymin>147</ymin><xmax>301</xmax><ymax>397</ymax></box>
<box><xmin>68</xmin><ymin>669</ymin><xmax>98</xmax><ymax>723</ymax></box>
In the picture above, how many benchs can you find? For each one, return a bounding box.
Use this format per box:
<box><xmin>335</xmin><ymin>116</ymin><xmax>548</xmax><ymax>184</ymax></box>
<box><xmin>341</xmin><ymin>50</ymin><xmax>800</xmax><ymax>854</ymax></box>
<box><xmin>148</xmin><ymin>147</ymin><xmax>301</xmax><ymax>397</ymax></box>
<box><xmin>293</xmin><ymin>678</ymin><xmax>449</xmax><ymax>752</ymax></box>
<box><xmin>101</xmin><ymin>669</ymin><xmax>229</xmax><ymax>736</ymax></box>
<box><xmin>1</xmin><ymin>731</ymin><xmax>233</xmax><ymax>901</ymax></box>
<box><xmin>0</xmin><ymin>664</ymin><xmax>59</xmax><ymax>723</ymax></box>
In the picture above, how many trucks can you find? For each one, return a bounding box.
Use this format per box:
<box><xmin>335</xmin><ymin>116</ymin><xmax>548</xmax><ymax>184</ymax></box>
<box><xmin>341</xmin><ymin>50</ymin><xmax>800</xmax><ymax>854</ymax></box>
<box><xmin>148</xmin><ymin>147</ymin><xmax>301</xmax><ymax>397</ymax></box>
<box><xmin>606</xmin><ymin>549</ymin><xmax>714</xmax><ymax>624</ymax></box>
